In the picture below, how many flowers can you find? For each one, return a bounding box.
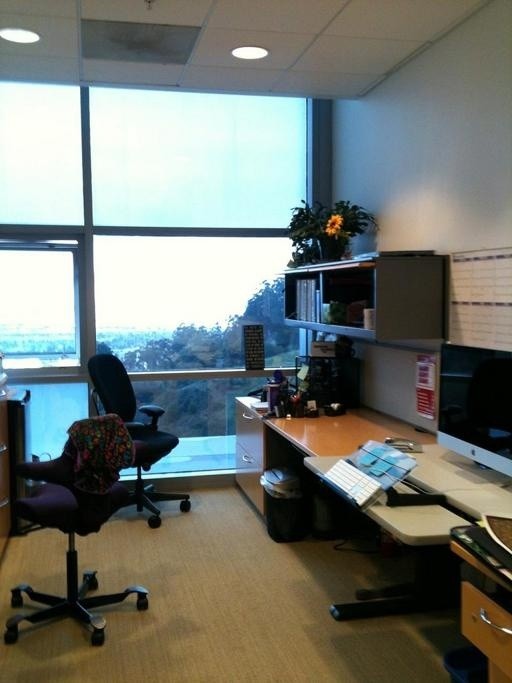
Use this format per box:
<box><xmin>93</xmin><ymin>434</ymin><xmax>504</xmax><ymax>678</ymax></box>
<box><xmin>288</xmin><ymin>201</ymin><xmax>379</xmax><ymax>236</ymax></box>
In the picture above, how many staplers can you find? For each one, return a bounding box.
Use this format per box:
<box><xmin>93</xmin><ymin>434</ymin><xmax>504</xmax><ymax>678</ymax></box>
<box><xmin>384</xmin><ymin>437</ymin><xmax>423</xmax><ymax>453</ymax></box>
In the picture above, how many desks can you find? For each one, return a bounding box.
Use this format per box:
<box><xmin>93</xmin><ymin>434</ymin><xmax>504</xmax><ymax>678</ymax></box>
<box><xmin>234</xmin><ymin>396</ymin><xmax>512</xmax><ymax>683</ymax></box>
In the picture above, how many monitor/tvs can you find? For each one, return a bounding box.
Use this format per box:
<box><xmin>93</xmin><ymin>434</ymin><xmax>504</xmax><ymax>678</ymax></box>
<box><xmin>437</xmin><ymin>343</ymin><xmax>512</xmax><ymax>487</ymax></box>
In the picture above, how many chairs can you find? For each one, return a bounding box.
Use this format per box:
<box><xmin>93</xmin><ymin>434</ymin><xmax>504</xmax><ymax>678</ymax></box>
<box><xmin>4</xmin><ymin>412</ymin><xmax>149</xmax><ymax>647</ymax></box>
<box><xmin>88</xmin><ymin>353</ymin><xmax>192</xmax><ymax>529</ymax></box>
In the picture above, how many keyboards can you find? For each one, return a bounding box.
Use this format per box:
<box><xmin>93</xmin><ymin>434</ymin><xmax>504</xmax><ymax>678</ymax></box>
<box><xmin>324</xmin><ymin>459</ymin><xmax>382</xmax><ymax>507</ymax></box>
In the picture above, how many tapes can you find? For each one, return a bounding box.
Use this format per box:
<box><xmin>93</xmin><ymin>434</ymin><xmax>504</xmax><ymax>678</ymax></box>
<box><xmin>330</xmin><ymin>403</ymin><xmax>341</xmax><ymax>411</ymax></box>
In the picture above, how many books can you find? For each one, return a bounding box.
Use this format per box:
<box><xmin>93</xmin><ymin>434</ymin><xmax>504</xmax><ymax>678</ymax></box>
<box><xmin>291</xmin><ymin>279</ymin><xmax>321</xmax><ymax>323</ymax></box>
<box><xmin>354</xmin><ymin>248</ymin><xmax>435</xmax><ymax>255</ymax></box>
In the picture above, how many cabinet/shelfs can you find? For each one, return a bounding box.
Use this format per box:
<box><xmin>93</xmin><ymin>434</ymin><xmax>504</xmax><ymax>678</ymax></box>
<box><xmin>285</xmin><ymin>255</ymin><xmax>447</xmax><ymax>352</ymax></box>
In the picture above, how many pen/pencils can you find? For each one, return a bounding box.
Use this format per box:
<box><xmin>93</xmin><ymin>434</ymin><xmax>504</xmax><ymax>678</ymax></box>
<box><xmin>297</xmin><ymin>390</ymin><xmax>303</xmax><ymax>399</ymax></box>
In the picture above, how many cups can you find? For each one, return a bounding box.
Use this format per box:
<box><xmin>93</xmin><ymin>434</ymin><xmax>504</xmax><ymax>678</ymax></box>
<box><xmin>363</xmin><ymin>309</ymin><xmax>376</xmax><ymax>330</ymax></box>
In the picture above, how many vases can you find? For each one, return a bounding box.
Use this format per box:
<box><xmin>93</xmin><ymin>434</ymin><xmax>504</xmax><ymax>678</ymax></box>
<box><xmin>312</xmin><ymin>233</ymin><xmax>352</xmax><ymax>262</ymax></box>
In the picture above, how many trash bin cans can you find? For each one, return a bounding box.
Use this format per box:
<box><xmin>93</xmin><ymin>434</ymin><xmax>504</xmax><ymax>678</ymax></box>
<box><xmin>260</xmin><ymin>467</ymin><xmax>348</xmax><ymax>543</ymax></box>
